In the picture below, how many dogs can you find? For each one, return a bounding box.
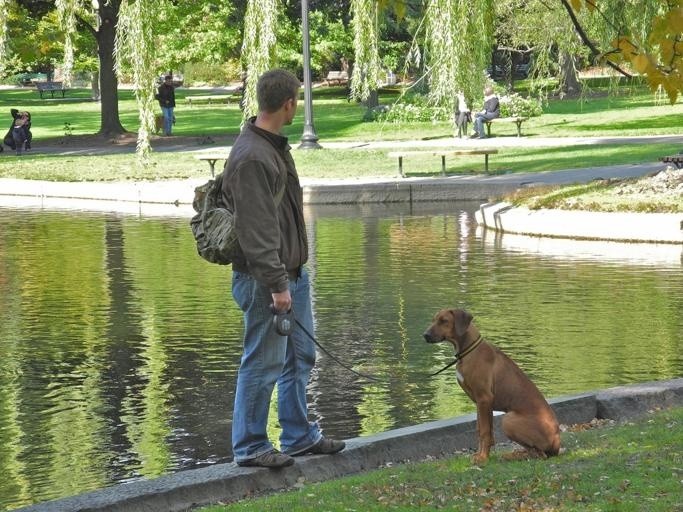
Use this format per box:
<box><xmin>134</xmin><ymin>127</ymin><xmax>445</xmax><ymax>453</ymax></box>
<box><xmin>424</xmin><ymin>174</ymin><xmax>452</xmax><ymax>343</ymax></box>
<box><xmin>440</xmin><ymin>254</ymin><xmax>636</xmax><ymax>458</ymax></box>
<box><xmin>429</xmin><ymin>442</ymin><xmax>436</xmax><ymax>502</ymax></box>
<box><xmin>151</xmin><ymin>115</ymin><xmax>176</xmax><ymax>134</ymax></box>
<box><xmin>423</xmin><ymin>307</ymin><xmax>561</xmax><ymax>465</ymax></box>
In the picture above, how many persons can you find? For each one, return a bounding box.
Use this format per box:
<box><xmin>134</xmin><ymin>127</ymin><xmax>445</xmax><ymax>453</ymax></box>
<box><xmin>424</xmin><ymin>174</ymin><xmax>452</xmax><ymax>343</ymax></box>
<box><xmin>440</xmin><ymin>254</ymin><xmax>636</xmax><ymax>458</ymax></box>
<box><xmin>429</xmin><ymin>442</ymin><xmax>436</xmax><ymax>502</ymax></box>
<box><xmin>468</xmin><ymin>85</ymin><xmax>500</xmax><ymax>139</ymax></box>
<box><xmin>2</xmin><ymin>108</ymin><xmax>32</xmax><ymax>151</ymax></box>
<box><xmin>13</xmin><ymin>120</ymin><xmax>24</xmax><ymax>154</ymax></box>
<box><xmin>226</xmin><ymin>70</ymin><xmax>348</xmax><ymax>471</ymax></box>
<box><xmin>157</xmin><ymin>75</ymin><xmax>174</xmax><ymax>136</ymax></box>
<box><xmin>452</xmin><ymin>89</ymin><xmax>472</xmax><ymax>137</ymax></box>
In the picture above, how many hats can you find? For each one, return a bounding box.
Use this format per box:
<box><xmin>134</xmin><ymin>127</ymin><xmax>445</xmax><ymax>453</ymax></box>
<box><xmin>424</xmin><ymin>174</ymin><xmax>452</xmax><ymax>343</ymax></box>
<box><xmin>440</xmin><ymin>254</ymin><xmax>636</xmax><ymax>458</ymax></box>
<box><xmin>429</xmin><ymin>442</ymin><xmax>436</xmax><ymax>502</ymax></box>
<box><xmin>15</xmin><ymin>119</ymin><xmax>25</xmax><ymax>126</ymax></box>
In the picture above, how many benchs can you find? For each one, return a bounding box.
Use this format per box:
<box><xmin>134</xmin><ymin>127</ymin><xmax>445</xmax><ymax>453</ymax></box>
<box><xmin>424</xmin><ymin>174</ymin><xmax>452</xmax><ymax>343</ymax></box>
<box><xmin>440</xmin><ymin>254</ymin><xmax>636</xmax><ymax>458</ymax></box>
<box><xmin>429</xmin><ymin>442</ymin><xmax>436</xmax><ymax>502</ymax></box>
<box><xmin>387</xmin><ymin>148</ymin><xmax>497</xmax><ymax>176</ymax></box>
<box><xmin>471</xmin><ymin>116</ymin><xmax>528</xmax><ymax>138</ymax></box>
<box><xmin>193</xmin><ymin>154</ymin><xmax>229</xmax><ymax>178</ymax></box>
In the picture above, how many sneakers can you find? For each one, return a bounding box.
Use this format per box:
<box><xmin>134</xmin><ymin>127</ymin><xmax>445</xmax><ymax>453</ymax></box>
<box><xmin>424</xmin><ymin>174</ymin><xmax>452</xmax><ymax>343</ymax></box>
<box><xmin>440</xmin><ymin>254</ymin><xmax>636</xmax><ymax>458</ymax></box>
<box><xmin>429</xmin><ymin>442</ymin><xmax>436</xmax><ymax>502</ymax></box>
<box><xmin>237</xmin><ymin>447</ymin><xmax>295</xmax><ymax>469</ymax></box>
<box><xmin>289</xmin><ymin>434</ymin><xmax>347</xmax><ymax>458</ymax></box>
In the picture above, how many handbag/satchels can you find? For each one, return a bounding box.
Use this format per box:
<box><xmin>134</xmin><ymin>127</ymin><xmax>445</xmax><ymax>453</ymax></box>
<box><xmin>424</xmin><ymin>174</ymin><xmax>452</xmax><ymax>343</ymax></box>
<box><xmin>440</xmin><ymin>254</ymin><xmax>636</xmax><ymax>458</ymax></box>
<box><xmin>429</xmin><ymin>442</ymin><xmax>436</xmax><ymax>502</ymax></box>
<box><xmin>189</xmin><ymin>171</ymin><xmax>238</xmax><ymax>265</ymax></box>
